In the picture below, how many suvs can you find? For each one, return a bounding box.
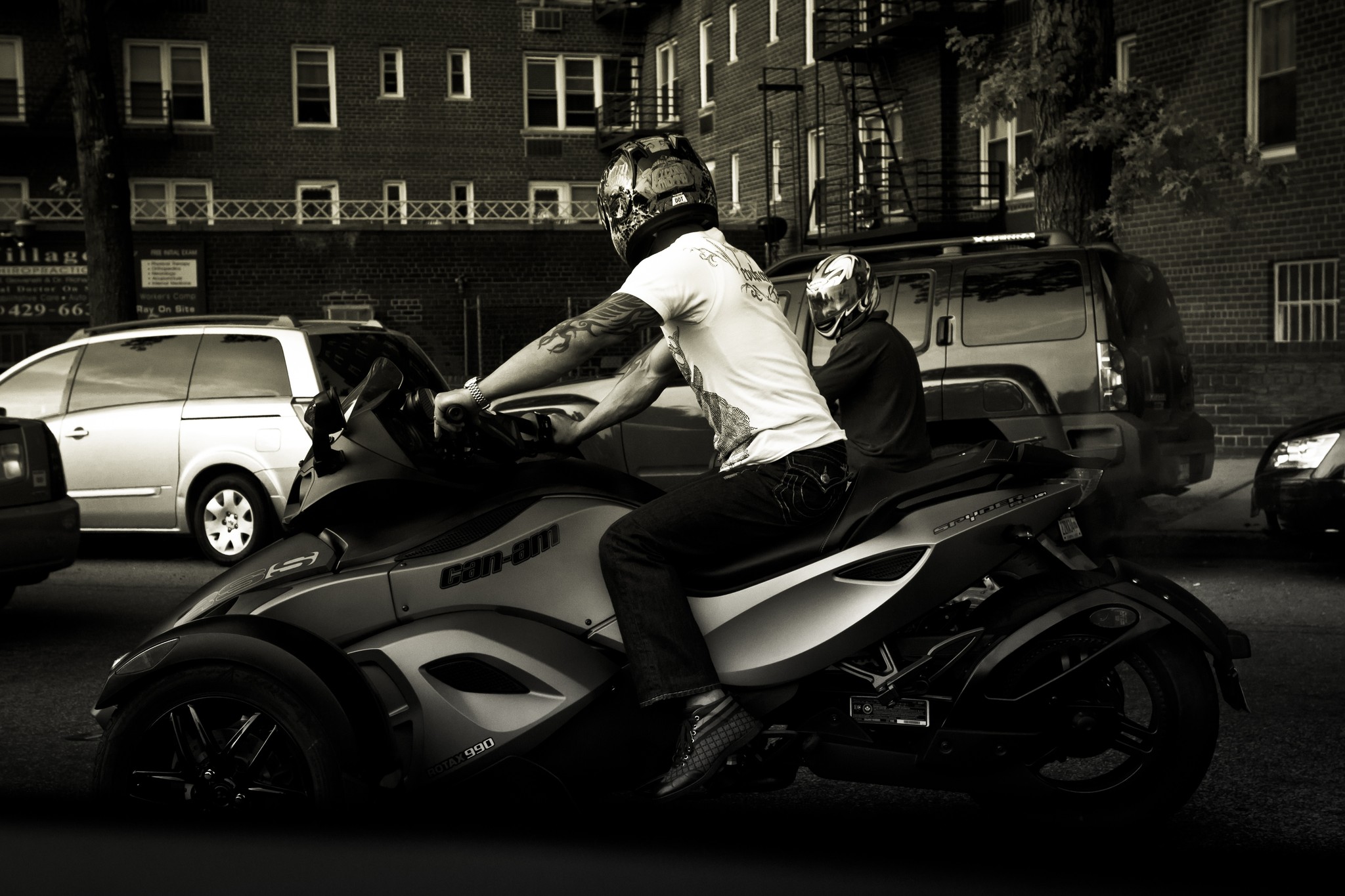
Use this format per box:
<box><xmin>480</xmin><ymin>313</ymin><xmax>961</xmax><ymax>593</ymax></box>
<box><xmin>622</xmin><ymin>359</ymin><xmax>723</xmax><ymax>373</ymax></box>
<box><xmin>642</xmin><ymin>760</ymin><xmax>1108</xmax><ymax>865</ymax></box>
<box><xmin>482</xmin><ymin>227</ymin><xmax>1218</xmax><ymax>503</ymax></box>
<box><xmin>0</xmin><ymin>309</ymin><xmax>453</xmax><ymax>567</ymax></box>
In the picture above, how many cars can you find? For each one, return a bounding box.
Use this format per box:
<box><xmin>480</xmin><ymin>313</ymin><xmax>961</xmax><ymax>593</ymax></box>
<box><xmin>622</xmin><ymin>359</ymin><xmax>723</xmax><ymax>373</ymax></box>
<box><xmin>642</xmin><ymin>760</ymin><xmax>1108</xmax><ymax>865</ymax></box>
<box><xmin>1248</xmin><ymin>413</ymin><xmax>1345</xmax><ymax>569</ymax></box>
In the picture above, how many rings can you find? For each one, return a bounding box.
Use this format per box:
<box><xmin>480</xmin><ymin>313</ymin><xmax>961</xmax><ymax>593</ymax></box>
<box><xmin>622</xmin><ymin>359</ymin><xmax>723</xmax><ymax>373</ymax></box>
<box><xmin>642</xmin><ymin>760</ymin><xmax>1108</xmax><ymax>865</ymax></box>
<box><xmin>433</xmin><ymin>416</ymin><xmax>436</xmax><ymax>420</ymax></box>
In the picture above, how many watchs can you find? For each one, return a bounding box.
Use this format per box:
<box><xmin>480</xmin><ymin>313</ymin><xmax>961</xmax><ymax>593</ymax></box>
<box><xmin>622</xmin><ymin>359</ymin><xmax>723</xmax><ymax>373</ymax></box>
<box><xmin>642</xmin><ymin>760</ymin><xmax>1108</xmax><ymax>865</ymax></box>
<box><xmin>463</xmin><ymin>376</ymin><xmax>490</xmax><ymax>410</ymax></box>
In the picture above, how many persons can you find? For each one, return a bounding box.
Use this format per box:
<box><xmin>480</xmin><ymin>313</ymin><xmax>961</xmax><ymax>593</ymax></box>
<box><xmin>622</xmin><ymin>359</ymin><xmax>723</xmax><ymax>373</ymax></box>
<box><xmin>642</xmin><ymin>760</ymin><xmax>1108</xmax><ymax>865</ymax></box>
<box><xmin>802</xmin><ymin>252</ymin><xmax>934</xmax><ymax>487</ymax></box>
<box><xmin>426</xmin><ymin>132</ymin><xmax>856</xmax><ymax>803</ymax></box>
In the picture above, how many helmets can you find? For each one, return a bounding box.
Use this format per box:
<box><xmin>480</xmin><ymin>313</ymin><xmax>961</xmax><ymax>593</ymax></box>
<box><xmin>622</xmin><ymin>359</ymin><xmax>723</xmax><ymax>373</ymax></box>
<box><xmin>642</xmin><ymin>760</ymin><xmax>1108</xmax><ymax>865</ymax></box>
<box><xmin>598</xmin><ymin>132</ymin><xmax>719</xmax><ymax>272</ymax></box>
<box><xmin>806</xmin><ymin>254</ymin><xmax>883</xmax><ymax>342</ymax></box>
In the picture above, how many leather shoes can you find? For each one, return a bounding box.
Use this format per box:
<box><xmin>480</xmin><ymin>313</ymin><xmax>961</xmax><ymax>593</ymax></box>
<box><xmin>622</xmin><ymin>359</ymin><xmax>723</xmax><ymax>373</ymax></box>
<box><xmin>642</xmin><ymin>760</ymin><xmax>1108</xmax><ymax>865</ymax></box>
<box><xmin>654</xmin><ymin>694</ymin><xmax>761</xmax><ymax>798</ymax></box>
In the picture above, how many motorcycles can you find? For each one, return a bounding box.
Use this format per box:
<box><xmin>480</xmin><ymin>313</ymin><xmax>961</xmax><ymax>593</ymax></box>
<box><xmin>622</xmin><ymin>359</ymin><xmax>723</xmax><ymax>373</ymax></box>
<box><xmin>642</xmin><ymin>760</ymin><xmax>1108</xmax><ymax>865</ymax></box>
<box><xmin>92</xmin><ymin>356</ymin><xmax>1259</xmax><ymax>836</ymax></box>
